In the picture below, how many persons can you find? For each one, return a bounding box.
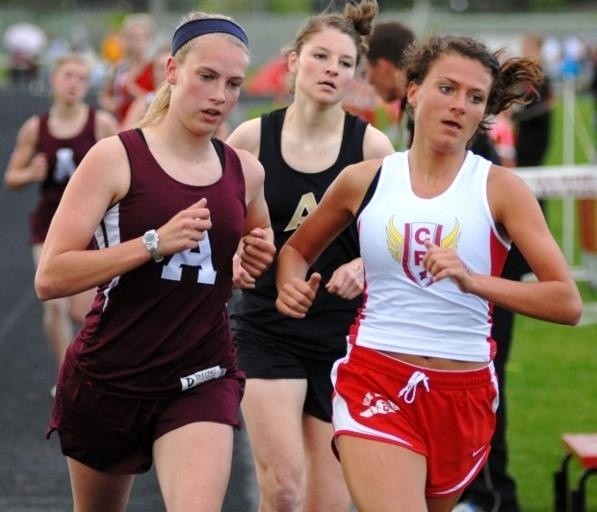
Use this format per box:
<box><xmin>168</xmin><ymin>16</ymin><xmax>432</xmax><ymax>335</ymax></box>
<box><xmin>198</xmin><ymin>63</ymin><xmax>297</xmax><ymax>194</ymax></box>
<box><xmin>274</xmin><ymin>35</ymin><xmax>583</xmax><ymax>512</ymax></box>
<box><xmin>360</xmin><ymin>19</ymin><xmax>541</xmax><ymax>512</ymax></box>
<box><xmin>224</xmin><ymin>0</ymin><xmax>396</xmax><ymax>512</ymax></box>
<box><xmin>2</xmin><ymin>55</ymin><xmax>125</xmax><ymax>402</ymax></box>
<box><xmin>487</xmin><ymin>33</ymin><xmax>554</xmax><ymax>223</ymax></box>
<box><xmin>4</xmin><ymin>12</ymin><xmax>171</xmax><ymax>131</ymax></box>
<box><xmin>33</xmin><ymin>9</ymin><xmax>278</xmax><ymax>512</ymax></box>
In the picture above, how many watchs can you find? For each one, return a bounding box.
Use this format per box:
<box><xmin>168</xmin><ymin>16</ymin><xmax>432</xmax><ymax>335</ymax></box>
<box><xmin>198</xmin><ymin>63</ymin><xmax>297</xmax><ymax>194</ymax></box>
<box><xmin>141</xmin><ymin>229</ymin><xmax>166</xmax><ymax>264</ymax></box>
